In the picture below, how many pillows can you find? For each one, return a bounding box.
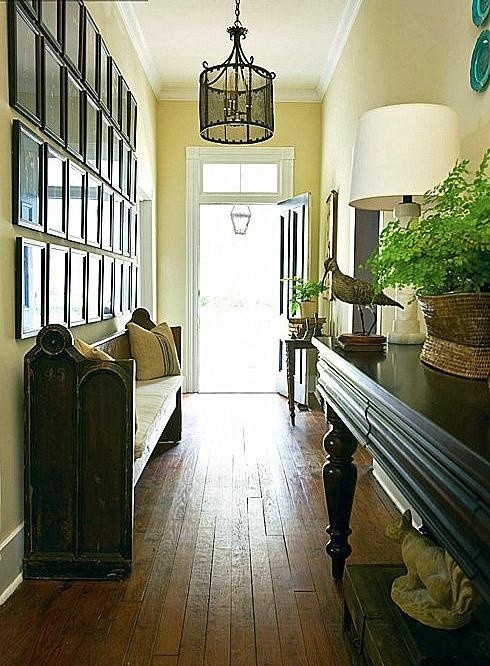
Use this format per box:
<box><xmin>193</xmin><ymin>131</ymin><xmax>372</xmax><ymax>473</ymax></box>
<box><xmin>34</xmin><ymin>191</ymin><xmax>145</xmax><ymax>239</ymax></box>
<box><xmin>128</xmin><ymin>320</ymin><xmax>182</xmax><ymax>381</ymax></box>
<box><xmin>74</xmin><ymin>336</ymin><xmax>139</xmax><ymax>435</ymax></box>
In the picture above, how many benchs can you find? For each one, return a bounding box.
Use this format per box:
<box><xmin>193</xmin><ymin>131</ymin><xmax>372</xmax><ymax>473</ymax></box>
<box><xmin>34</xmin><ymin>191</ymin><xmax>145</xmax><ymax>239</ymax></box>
<box><xmin>22</xmin><ymin>306</ymin><xmax>186</xmax><ymax>585</ymax></box>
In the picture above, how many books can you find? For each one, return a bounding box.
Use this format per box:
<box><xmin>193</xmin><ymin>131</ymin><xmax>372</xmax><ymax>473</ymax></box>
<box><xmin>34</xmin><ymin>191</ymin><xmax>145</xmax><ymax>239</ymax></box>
<box><xmin>334</xmin><ymin>332</ymin><xmax>388</xmax><ymax>353</ymax></box>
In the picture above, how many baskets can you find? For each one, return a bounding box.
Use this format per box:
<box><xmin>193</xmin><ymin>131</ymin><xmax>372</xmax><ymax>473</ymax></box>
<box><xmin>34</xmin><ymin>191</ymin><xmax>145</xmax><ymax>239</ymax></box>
<box><xmin>415</xmin><ymin>293</ymin><xmax>490</xmax><ymax>380</ymax></box>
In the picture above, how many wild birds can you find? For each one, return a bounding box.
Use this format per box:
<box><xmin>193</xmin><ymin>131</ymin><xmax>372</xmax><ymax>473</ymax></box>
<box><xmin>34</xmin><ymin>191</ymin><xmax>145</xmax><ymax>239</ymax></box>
<box><xmin>320</xmin><ymin>257</ymin><xmax>404</xmax><ymax>336</ymax></box>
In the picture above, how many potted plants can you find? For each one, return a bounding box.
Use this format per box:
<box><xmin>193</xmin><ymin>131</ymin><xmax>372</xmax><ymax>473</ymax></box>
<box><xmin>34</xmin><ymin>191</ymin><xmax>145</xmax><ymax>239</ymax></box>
<box><xmin>357</xmin><ymin>147</ymin><xmax>490</xmax><ymax>380</ymax></box>
<box><xmin>277</xmin><ymin>276</ymin><xmax>329</xmax><ymax>336</ymax></box>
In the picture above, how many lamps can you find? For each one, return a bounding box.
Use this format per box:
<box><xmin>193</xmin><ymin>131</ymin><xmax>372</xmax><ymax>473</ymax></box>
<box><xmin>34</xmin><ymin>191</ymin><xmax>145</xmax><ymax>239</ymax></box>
<box><xmin>349</xmin><ymin>101</ymin><xmax>462</xmax><ymax>344</ymax></box>
<box><xmin>195</xmin><ymin>0</ymin><xmax>276</xmax><ymax>145</ymax></box>
<box><xmin>228</xmin><ymin>162</ymin><xmax>252</xmax><ymax>234</ymax></box>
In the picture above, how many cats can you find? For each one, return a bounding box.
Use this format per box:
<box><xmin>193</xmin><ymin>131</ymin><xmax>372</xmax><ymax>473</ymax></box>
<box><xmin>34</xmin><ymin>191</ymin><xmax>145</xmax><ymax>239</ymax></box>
<box><xmin>384</xmin><ymin>509</ymin><xmax>453</xmax><ymax>611</ymax></box>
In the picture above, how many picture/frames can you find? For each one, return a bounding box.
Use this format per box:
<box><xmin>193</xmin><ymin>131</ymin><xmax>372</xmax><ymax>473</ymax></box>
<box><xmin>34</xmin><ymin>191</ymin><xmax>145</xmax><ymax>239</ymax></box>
<box><xmin>8</xmin><ymin>0</ymin><xmax>139</xmax><ymax>340</ymax></box>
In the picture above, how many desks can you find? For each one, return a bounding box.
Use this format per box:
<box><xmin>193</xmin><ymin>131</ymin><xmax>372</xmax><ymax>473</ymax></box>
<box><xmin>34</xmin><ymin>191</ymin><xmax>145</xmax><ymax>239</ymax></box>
<box><xmin>309</xmin><ymin>335</ymin><xmax>488</xmax><ymax>665</ymax></box>
<box><xmin>278</xmin><ymin>336</ymin><xmax>331</xmax><ymax>427</ymax></box>
